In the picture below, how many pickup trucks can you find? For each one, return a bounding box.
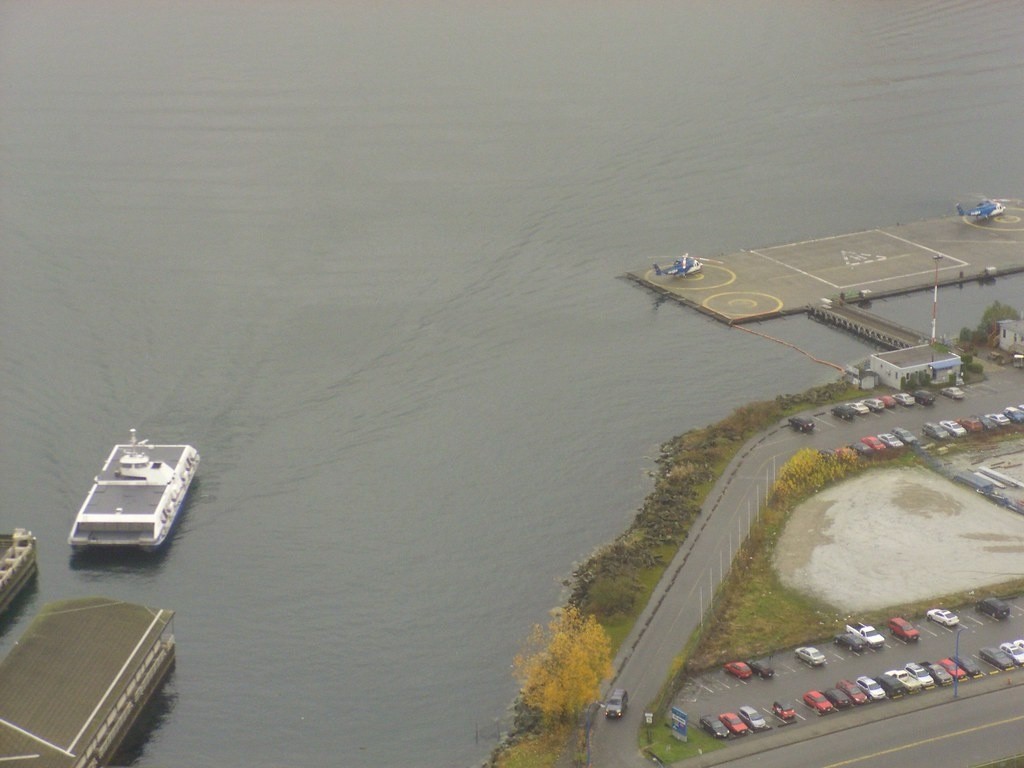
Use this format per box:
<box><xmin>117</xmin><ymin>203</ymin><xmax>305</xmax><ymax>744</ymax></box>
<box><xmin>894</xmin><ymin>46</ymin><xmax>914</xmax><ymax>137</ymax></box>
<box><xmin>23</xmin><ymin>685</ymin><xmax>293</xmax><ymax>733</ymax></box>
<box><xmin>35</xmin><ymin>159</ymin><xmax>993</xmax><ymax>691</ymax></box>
<box><xmin>919</xmin><ymin>662</ymin><xmax>954</xmax><ymax>683</ymax></box>
<box><xmin>885</xmin><ymin>670</ymin><xmax>924</xmax><ymax>694</ymax></box>
<box><xmin>846</xmin><ymin>621</ymin><xmax>886</xmax><ymax>649</ymax></box>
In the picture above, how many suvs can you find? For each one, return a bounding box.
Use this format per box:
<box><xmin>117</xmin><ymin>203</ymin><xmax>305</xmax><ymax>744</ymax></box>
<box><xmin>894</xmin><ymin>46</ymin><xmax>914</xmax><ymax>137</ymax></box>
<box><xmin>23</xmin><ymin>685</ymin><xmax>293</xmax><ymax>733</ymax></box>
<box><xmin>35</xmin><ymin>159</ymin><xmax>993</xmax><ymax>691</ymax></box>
<box><xmin>976</xmin><ymin>597</ymin><xmax>1010</xmax><ymax>619</ymax></box>
<box><xmin>836</xmin><ymin>679</ymin><xmax>868</xmax><ymax>706</ymax></box>
<box><xmin>605</xmin><ymin>690</ymin><xmax>629</xmax><ymax>720</ymax></box>
<box><xmin>888</xmin><ymin>617</ymin><xmax>920</xmax><ymax>642</ymax></box>
<box><xmin>737</xmin><ymin>706</ymin><xmax>767</xmax><ymax>731</ymax></box>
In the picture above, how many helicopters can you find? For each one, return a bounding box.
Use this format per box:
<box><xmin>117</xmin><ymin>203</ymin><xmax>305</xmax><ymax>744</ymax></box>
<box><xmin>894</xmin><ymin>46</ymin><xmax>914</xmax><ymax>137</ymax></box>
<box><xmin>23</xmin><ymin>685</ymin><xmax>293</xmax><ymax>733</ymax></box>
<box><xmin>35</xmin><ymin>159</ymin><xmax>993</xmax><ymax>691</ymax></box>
<box><xmin>955</xmin><ymin>198</ymin><xmax>1008</xmax><ymax>223</ymax></box>
<box><xmin>653</xmin><ymin>252</ymin><xmax>724</xmax><ymax>281</ymax></box>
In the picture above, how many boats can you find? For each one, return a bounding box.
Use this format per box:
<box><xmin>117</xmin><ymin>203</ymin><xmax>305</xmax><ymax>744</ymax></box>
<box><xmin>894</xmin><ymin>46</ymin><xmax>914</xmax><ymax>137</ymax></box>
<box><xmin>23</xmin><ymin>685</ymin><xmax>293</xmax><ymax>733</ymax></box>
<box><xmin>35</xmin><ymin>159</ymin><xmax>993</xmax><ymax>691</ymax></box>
<box><xmin>67</xmin><ymin>427</ymin><xmax>201</xmax><ymax>559</ymax></box>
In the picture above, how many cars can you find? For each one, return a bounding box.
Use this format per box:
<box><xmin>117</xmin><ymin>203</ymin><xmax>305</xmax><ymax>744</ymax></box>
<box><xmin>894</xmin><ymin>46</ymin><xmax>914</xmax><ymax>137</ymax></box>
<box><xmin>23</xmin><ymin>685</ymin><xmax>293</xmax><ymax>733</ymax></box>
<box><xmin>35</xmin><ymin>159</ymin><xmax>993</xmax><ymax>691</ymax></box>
<box><xmin>719</xmin><ymin>712</ymin><xmax>748</xmax><ymax>737</ymax></box>
<box><xmin>725</xmin><ymin>662</ymin><xmax>752</xmax><ymax>678</ymax></box>
<box><xmin>872</xmin><ymin>674</ymin><xmax>906</xmax><ymax>698</ymax></box>
<box><xmin>856</xmin><ymin>676</ymin><xmax>886</xmax><ymax>700</ymax></box>
<box><xmin>818</xmin><ymin>688</ymin><xmax>851</xmax><ymax>708</ymax></box>
<box><xmin>773</xmin><ymin>700</ymin><xmax>795</xmax><ymax>719</ymax></box>
<box><xmin>701</xmin><ymin>714</ymin><xmax>729</xmax><ymax>740</ymax></box>
<box><xmin>803</xmin><ymin>691</ymin><xmax>833</xmax><ymax>713</ymax></box>
<box><xmin>979</xmin><ymin>647</ymin><xmax>1014</xmax><ymax>669</ymax></box>
<box><xmin>795</xmin><ymin>646</ymin><xmax>826</xmax><ymax>666</ymax></box>
<box><xmin>949</xmin><ymin>655</ymin><xmax>982</xmax><ymax>676</ymax></box>
<box><xmin>783</xmin><ymin>385</ymin><xmax>1024</xmax><ymax>463</ymax></box>
<box><xmin>746</xmin><ymin>659</ymin><xmax>774</xmax><ymax>678</ymax></box>
<box><xmin>1014</xmin><ymin>640</ymin><xmax>1024</xmax><ymax>650</ymax></box>
<box><xmin>904</xmin><ymin>663</ymin><xmax>935</xmax><ymax>688</ymax></box>
<box><xmin>927</xmin><ymin>609</ymin><xmax>960</xmax><ymax>628</ymax></box>
<box><xmin>999</xmin><ymin>643</ymin><xmax>1024</xmax><ymax>666</ymax></box>
<box><xmin>834</xmin><ymin>633</ymin><xmax>865</xmax><ymax>652</ymax></box>
<box><xmin>936</xmin><ymin>658</ymin><xmax>966</xmax><ymax>679</ymax></box>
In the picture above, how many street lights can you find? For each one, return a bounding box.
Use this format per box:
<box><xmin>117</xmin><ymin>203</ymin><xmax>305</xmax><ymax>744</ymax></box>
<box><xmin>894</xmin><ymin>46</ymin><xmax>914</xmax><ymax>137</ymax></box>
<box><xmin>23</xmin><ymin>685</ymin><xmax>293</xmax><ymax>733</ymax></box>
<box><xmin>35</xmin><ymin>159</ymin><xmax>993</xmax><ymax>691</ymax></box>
<box><xmin>932</xmin><ymin>253</ymin><xmax>943</xmax><ymax>342</ymax></box>
